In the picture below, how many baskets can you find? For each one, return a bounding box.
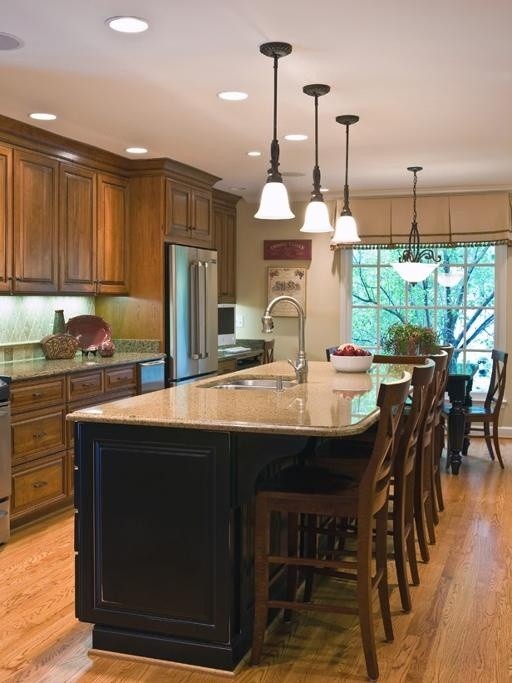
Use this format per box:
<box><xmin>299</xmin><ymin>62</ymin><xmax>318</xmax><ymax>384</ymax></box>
<box><xmin>39</xmin><ymin>331</ymin><xmax>81</xmax><ymax>361</ymax></box>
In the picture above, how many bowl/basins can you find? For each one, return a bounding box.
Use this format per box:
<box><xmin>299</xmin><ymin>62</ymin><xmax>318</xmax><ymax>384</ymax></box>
<box><xmin>330</xmin><ymin>353</ymin><xmax>375</xmax><ymax>372</ymax></box>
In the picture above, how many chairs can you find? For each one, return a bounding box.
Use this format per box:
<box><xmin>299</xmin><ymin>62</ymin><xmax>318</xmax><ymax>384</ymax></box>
<box><xmin>262</xmin><ymin>338</ymin><xmax>275</xmax><ymax>364</ymax></box>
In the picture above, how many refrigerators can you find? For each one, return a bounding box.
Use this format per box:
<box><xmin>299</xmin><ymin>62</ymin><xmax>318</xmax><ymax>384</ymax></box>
<box><xmin>166</xmin><ymin>243</ymin><xmax>219</xmax><ymax>387</ymax></box>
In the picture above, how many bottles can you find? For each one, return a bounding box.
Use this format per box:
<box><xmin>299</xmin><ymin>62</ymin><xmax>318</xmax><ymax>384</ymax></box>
<box><xmin>97</xmin><ymin>333</ymin><xmax>115</xmax><ymax>357</ymax></box>
<box><xmin>53</xmin><ymin>308</ymin><xmax>66</xmax><ymax>333</ymax></box>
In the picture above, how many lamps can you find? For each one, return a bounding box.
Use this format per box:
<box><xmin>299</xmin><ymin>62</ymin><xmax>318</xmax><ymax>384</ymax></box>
<box><xmin>389</xmin><ymin>165</ymin><xmax>440</xmax><ymax>287</ymax></box>
<box><xmin>300</xmin><ymin>84</ymin><xmax>334</xmax><ymax>234</ymax></box>
<box><xmin>331</xmin><ymin>114</ymin><xmax>361</xmax><ymax>246</ymax></box>
<box><xmin>437</xmin><ymin>272</ymin><xmax>463</xmax><ymax>290</ymax></box>
<box><xmin>253</xmin><ymin>41</ymin><xmax>296</xmax><ymax>221</ymax></box>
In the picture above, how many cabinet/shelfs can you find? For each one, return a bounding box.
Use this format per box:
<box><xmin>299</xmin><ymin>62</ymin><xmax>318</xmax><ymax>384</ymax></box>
<box><xmin>1</xmin><ymin>142</ymin><xmax>242</xmax><ymax>304</ymax></box>
<box><xmin>9</xmin><ymin>363</ymin><xmax>150</xmax><ymax>530</ymax></box>
<box><xmin>218</xmin><ymin>352</ymin><xmax>264</xmax><ymax>374</ymax></box>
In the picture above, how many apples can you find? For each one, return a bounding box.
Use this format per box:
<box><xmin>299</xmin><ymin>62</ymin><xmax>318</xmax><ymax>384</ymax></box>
<box><xmin>334</xmin><ymin>345</ymin><xmax>371</xmax><ymax>356</ymax></box>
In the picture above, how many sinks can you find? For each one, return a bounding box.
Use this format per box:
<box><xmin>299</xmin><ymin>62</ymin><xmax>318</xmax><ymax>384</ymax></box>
<box><xmin>210</xmin><ymin>371</ymin><xmax>294</xmax><ymax>387</ymax></box>
<box><xmin>195</xmin><ymin>384</ymin><xmax>292</xmax><ymax>394</ymax></box>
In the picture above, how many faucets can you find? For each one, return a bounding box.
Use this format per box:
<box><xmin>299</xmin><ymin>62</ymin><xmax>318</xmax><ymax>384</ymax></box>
<box><xmin>259</xmin><ymin>296</ymin><xmax>310</xmax><ymax>381</ymax></box>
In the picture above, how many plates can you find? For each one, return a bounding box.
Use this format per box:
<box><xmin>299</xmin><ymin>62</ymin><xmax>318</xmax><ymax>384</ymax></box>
<box><xmin>65</xmin><ymin>314</ymin><xmax>111</xmax><ymax>351</ymax></box>
